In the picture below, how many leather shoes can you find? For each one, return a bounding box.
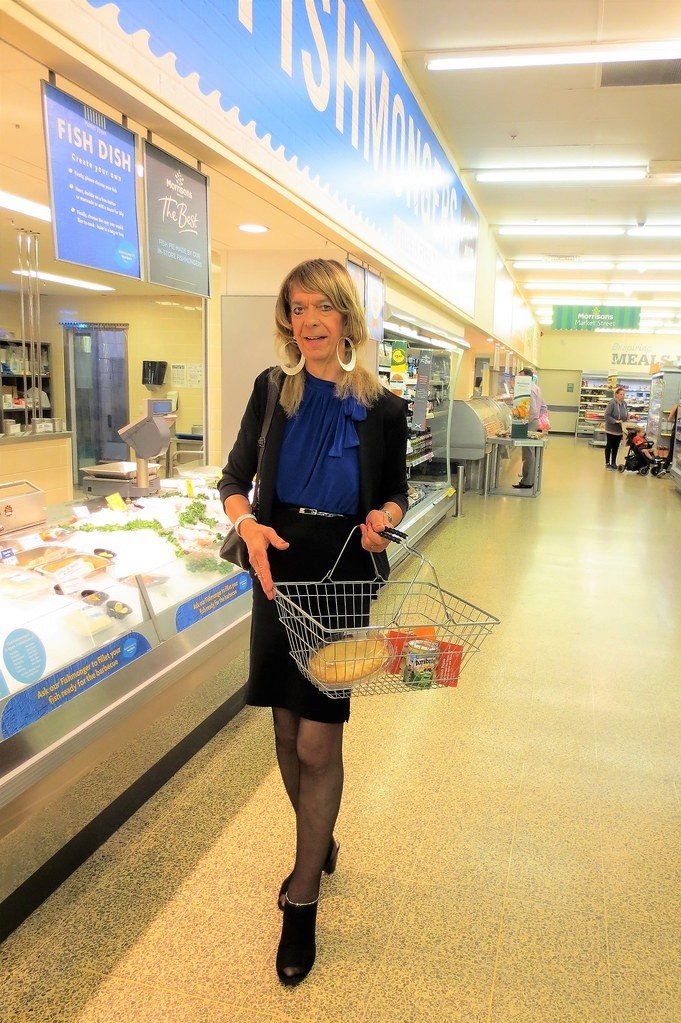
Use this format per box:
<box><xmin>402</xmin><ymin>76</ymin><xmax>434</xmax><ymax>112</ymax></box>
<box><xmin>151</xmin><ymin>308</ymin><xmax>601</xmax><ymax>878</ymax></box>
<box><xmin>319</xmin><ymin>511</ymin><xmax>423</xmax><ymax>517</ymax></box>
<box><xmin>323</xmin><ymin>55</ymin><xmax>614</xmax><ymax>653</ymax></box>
<box><xmin>512</xmin><ymin>483</ymin><xmax>532</xmax><ymax>488</ymax></box>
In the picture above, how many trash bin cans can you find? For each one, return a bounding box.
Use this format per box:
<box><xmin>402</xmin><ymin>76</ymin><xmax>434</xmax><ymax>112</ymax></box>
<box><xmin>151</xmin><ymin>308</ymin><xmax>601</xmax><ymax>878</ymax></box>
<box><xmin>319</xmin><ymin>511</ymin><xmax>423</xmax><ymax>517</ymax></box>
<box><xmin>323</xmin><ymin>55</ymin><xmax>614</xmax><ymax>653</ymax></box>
<box><xmin>78</xmin><ymin>415</ymin><xmax>171</xmax><ymax>497</ymax></box>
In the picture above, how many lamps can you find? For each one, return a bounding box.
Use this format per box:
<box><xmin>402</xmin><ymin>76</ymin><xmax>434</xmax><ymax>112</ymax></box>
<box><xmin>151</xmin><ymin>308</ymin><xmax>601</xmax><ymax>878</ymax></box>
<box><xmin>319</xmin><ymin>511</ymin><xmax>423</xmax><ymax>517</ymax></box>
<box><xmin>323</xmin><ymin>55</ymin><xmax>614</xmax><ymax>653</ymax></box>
<box><xmin>424</xmin><ymin>41</ymin><xmax>679</xmax><ymax>71</ymax></box>
<box><xmin>472</xmin><ymin>160</ymin><xmax>652</xmax><ymax>184</ymax></box>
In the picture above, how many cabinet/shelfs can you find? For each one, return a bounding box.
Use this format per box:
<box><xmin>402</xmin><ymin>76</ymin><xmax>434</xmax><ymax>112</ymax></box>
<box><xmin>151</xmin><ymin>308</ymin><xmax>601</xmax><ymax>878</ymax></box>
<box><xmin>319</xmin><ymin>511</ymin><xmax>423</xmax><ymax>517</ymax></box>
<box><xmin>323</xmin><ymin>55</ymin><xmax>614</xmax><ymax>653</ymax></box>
<box><xmin>574</xmin><ymin>375</ymin><xmax>681</xmax><ymax>490</ymax></box>
<box><xmin>0</xmin><ymin>337</ymin><xmax>56</xmax><ymax>434</ymax></box>
<box><xmin>377</xmin><ymin>326</ymin><xmax>451</xmax><ymax>489</ymax></box>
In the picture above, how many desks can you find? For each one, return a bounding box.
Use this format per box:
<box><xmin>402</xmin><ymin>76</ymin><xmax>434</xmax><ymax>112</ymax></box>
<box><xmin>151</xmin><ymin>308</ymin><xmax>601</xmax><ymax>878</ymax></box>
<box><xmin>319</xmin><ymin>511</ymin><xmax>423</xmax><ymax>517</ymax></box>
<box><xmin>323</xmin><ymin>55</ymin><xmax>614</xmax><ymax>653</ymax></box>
<box><xmin>484</xmin><ymin>432</ymin><xmax>547</xmax><ymax>497</ymax></box>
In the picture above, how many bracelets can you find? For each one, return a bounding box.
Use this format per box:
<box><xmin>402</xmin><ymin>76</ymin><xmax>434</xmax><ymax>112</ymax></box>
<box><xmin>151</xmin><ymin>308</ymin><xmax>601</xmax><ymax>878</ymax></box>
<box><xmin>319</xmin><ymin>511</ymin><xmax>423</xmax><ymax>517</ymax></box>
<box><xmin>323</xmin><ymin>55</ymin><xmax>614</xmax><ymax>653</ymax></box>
<box><xmin>234</xmin><ymin>513</ymin><xmax>257</xmax><ymax>537</ymax></box>
<box><xmin>381</xmin><ymin>509</ymin><xmax>393</xmax><ymax>526</ymax></box>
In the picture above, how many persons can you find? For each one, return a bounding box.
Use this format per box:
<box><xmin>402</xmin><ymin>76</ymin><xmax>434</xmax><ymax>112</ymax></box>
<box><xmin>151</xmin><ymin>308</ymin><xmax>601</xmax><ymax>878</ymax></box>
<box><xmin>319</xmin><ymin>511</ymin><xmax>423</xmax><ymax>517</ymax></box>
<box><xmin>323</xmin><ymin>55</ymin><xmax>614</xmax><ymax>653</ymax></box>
<box><xmin>632</xmin><ymin>427</ymin><xmax>660</xmax><ymax>460</ymax></box>
<box><xmin>657</xmin><ymin>403</ymin><xmax>679</xmax><ymax>478</ymax></box>
<box><xmin>603</xmin><ymin>388</ymin><xmax>631</xmax><ymax>471</ymax></box>
<box><xmin>512</xmin><ymin>368</ymin><xmax>542</xmax><ymax>488</ymax></box>
<box><xmin>217</xmin><ymin>257</ymin><xmax>408</xmax><ymax>988</ymax></box>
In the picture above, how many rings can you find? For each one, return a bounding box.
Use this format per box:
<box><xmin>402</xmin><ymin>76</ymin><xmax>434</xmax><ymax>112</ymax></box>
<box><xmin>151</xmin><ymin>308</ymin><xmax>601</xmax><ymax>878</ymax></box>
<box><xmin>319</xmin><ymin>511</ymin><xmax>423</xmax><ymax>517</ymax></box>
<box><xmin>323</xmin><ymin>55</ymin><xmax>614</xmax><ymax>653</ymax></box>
<box><xmin>371</xmin><ymin>545</ymin><xmax>374</xmax><ymax>549</ymax></box>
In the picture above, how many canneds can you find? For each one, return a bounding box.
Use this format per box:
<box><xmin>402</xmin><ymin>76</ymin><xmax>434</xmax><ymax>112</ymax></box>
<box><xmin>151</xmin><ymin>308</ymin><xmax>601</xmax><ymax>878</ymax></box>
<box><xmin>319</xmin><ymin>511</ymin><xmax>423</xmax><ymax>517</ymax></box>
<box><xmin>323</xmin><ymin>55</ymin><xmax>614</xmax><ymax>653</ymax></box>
<box><xmin>403</xmin><ymin>640</ymin><xmax>439</xmax><ymax>689</ymax></box>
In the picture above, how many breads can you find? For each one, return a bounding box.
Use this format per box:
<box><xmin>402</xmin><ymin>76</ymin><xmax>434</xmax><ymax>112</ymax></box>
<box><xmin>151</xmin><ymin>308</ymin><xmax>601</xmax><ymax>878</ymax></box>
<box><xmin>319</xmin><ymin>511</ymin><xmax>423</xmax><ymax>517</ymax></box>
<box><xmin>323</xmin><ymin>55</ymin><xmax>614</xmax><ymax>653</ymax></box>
<box><xmin>309</xmin><ymin>637</ymin><xmax>389</xmax><ymax>682</ymax></box>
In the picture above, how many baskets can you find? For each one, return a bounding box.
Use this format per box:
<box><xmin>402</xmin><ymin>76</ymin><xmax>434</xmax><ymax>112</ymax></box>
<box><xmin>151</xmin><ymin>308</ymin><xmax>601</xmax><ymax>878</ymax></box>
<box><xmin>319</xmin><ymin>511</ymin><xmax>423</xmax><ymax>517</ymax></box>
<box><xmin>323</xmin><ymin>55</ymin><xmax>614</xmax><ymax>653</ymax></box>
<box><xmin>272</xmin><ymin>525</ymin><xmax>501</xmax><ymax>699</ymax></box>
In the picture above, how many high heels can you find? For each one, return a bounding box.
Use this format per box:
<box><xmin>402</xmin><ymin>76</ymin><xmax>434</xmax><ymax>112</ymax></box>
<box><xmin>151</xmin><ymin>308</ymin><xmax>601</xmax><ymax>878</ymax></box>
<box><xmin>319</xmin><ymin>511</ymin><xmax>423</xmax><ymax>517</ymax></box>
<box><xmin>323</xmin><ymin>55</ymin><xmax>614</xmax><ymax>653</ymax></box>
<box><xmin>278</xmin><ymin>836</ymin><xmax>340</xmax><ymax>910</ymax></box>
<box><xmin>276</xmin><ymin>891</ymin><xmax>320</xmax><ymax>985</ymax></box>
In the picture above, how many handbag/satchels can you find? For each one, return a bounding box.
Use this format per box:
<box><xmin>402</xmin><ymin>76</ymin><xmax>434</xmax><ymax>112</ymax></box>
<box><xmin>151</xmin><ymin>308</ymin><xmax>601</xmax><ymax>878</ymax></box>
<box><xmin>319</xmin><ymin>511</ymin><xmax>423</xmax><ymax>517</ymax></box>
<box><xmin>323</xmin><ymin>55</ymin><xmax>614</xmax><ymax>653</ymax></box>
<box><xmin>219</xmin><ymin>501</ymin><xmax>261</xmax><ymax>571</ymax></box>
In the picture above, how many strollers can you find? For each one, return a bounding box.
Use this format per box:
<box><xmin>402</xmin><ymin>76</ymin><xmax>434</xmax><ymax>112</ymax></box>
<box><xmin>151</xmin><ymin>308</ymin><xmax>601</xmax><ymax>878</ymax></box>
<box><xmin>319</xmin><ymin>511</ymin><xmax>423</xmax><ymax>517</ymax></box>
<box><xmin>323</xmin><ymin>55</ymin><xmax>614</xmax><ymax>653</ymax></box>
<box><xmin>617</xmin><ymin>418</ymin><xmax>667</xmax><ymax>477</ymax></box>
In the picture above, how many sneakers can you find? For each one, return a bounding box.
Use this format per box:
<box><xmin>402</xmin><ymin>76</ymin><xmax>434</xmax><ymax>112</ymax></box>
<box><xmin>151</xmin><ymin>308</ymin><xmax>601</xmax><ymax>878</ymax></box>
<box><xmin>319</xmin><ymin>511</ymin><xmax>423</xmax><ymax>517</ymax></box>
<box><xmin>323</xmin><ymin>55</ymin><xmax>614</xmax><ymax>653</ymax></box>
<box><xmin>610</xmin><ymin>464</ymin><xmax>618</xmax><ymax>470</ymax></box>
<box><xmin>657</xmin><ymin>470</ymin><xmax>666</xmax><ymax>478</ymax></box>
<box><xmin>606</xmin><ymin>464</ymin><xmax>613</xmax><ymax>471</ymax></box>
